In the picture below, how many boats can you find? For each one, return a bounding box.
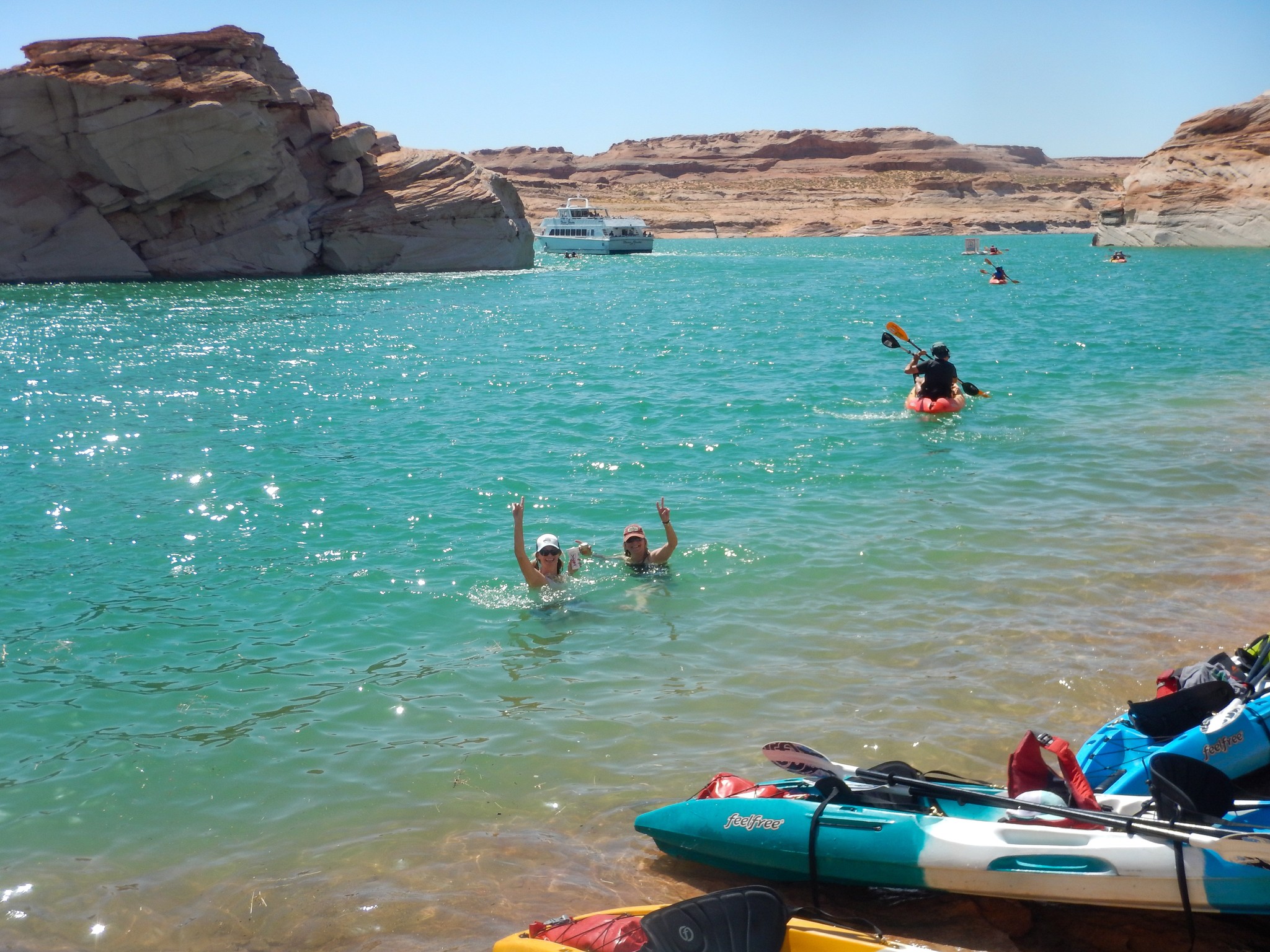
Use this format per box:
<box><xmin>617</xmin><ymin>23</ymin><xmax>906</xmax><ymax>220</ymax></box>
<box><xmin>534</xmin><ymin>195</ymin><xmax>657</xmax><ymax>255</ymax></box>
<box><xmin>1060</xmin><ymin>631</ymin><xmax>1270</xmax><ymax>797</ymax></box>
<box><xmin>905</xmin><ymin>381</ymin><xmax>967</xmax><ymax>411</ymax></box>
<box><xmin>1111</xmin><ymin>257</ymin><xmax>1127</xmax><ymax>263</ymax></box>
<box><xmin>631</xmin><ymin>758</ymin><xmax>1270</xmax><ymax>922</ymax></box>
<box><xmin>489</xmin><ymin>885</ymin><xmax>983</xmax><ymax>952</ymax></box>
<box><xmin>989</xmin><ymin>275</ymin><xmax>1008</xmax><ymax>284</ymax></box>
<box><xmin>989</xmin><ymin>251</ymin><xmax>1003</xmax><ymax>255</ymax></box>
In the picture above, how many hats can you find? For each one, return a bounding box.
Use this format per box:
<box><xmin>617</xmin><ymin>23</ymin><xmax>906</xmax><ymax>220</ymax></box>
<box><xmin>929</xmin><ymin>342</ymin><xmax>950</xmax><ymax>359</ymax></box>
<box><xmin>995</xmin><ymin>247</ymin><xmax>997</xmax><ymax>249</ymax></box>
<box><xmin>623</xmin><ymin>524</ymin><xmax>645</xmax><ymax>542</ymax></box>
<box><xmin>999</xmin><ymin>266</ymin><xmax>1003</xmax><ymax>269</ymax></box>
<box><xmin>1115</xmin><ymin>251</ymin><xmax>1118</xmax><ymax>253</ymax></box>
<box><xmin>991</xmin><ymin>245</ymin><xmax>994</xmax><ymax>247</ymax></box>
<box><xmin>1119</xmin><ymin>251</ymin><xmax>1122</xmax><ymax>252</ymax></box>
<box><xmin>536</xmin><ymin>533</ymin><xmax>560</xmax><ymax>552</ymax></box>
<box><xmin>995</xmin><ymin>267</ymin><xmax>1000</xmax><ymax>270</ymax></box>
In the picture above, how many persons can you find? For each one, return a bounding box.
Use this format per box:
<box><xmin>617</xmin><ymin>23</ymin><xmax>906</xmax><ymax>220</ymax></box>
<box><xmin>1111</xmin><ymin>251</ymin><xmax>1125</xmax><ymax>260</ymax></box>
<box><xmin>511</xmin><ymin>496</ymin><xmax>583</xmax><ymax>591</ymax></box>
<box><xmin>989</xmin><ymin>245</ymin><xmax>997</xmax><ymax>253</ymax></box>
<box><xmin>575</xmin><ymin>496</ymin><xmax>678</xmax><ymax>578</ymax></box>
<box><xmin>904</xmin><ymin>342</ymin><xmax>958</xmax><ymax>402</ymax></box>
<box><xmin>991</xmin><ymin>265</ymin><xmax>1007</xmax><ymax>281</ymax></box>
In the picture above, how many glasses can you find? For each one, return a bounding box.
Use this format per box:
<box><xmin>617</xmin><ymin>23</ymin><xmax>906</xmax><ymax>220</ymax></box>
<box><xmin>540</xmin><ymin>549</ymin><xmax>559</xmax><ymax>556</ymax></box>
<box><xmin>626</xmin><ymin>537</ymin><xmax>643</xmax><ymax>543</ymax></box>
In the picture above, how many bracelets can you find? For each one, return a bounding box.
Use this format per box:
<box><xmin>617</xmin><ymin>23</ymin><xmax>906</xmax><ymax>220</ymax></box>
<box><xmin>662</xmin><ymin>519</ymin><xmax>670</xmax><ymax>524</ymax></box>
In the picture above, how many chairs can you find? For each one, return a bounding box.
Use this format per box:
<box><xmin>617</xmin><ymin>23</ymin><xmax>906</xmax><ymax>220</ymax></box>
<box><xmin>1127</xmin><ymin>681</ymin><xmax>1237</xmax><ymax>742</ymax></box>
<box><xmin>918</xmin><ymin>377</ymin><xmax>952</xmax><ymax>399</ymax></box>
<box><xmin>814</xmin><ymin>760</ymin><xmax>925</xmax><ymax>817</ymax></box>
<box><xmin>641</xmin><ymin>883</ymin><xmax>788</xmax><ymax>952</ymax></box>
<box><xmin>1149</xmin><ymin>752</ymin><xmax>1236</xmax><ymax>832</ymax></box>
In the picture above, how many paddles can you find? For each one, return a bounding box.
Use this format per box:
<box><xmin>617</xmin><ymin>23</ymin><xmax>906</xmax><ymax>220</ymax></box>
<box><xmin>886</xmin><ymin>321</ymin><xmax>990</xmax><ymax>398</ymax></box>
<box><xmin>1199</xmin><ymin>640</ymin><xmax>1270</xmax><ymax>735</ymax></box>
<box><xmin>985</xmin><ymin>249</ymin><xmax>1009</xmax><ymax>251</ymax></box>
<box><xmin>1105</xmin><ymin>255</ymin><xmax>1113</xmax><ymax>258</ymax></box>
<box><xmin>881</xmin><ymin>332</ymin><xmax>980</xmax><ymax>397</ymax></box>
<box><xmin>761</xmin><ymin>741</ymin><xmax>1270</xmax><ymax>872</ymax></box>
<box><xmin>985</xmin><ymin>258</ymin><xmax>1020</xmax><ymax>284</ymax></box>
<box><xmin>980</xmin><ymin>269</ymin><xmax>1019</xmax><ymax>284</ymax></box>
<box><xmin>1109</xmin><ymin>248</ymin><xmax>1131</xmax><ymax>258</ymax></box>
<box><xmin>984</xmin><ymin>247</ymin><xmax>991</xmax><ymax>251</ymax></box>
